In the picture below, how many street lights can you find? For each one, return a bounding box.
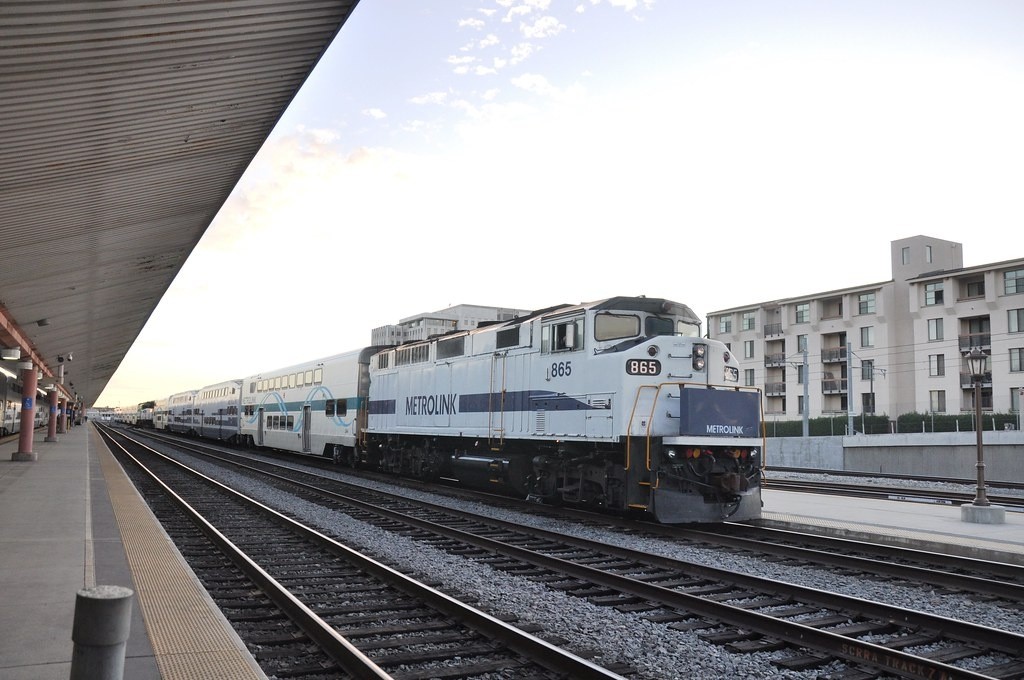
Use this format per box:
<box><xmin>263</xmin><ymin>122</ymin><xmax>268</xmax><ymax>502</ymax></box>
<box><xmin>964</xmin><ymin>345</ymin><xmax>992</xmax><ymax>505</ymax></box>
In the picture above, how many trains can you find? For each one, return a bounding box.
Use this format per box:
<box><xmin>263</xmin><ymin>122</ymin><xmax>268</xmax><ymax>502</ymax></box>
<box><xmin>102</xmin><ymin>295</ymin><xmax>764</xmax><ymax>525</ymax></box>
<box><xmin>0</xmin><ymin>372</ymin><xmax>61</xmax><ymax>439</ymax></box>
